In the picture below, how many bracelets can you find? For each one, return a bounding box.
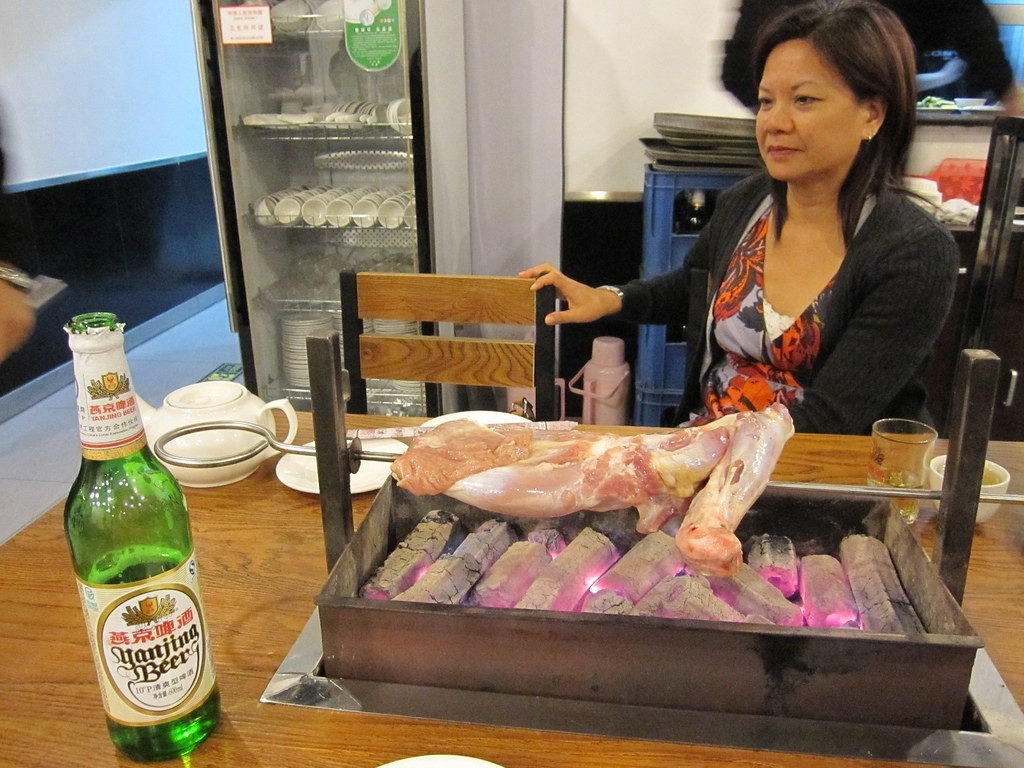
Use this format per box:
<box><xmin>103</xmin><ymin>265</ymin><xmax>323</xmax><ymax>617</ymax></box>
<box><xmin>597</xmin><ymin>284</ymin><xmax>625</xmax><ymax>300</ymax></box>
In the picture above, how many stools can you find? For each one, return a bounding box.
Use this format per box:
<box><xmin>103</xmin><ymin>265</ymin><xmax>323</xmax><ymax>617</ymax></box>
<box><xmin>632</xmin><ymin>162</ymin><xmax>747</xmax><ymax>427</ymax></box>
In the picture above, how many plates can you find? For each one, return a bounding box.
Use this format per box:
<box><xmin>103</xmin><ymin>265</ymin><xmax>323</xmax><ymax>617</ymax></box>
<box><xmin>280</xmin><ymin>312</ymin><xmax>427</xmax><ymax>395</ymax></box>
<box><xmin>916</xmin><ymin>105</ymin><xmax>960</xmax><ymax>113</ymax></box>
<box><xmin>957</xmin><ymin>106</ymin><xmax>1006</xmax><ymax>114</ymax></box>
<box><xmin>276</xmin><ymin>434</ymin><xmax>411</xmax><ymax>495</ymax></box>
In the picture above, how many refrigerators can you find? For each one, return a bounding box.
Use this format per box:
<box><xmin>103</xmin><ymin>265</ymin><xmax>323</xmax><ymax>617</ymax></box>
<box><xmin>189</xmin><ymin>0</ymin><xmax>472</xmax><ymax>418</ymax></box>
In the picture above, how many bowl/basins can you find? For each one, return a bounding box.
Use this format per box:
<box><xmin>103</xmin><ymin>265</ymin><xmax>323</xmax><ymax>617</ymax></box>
<box><xmin>234</xmin><ymin>0</ymin><xmax>351</xmax><ymax>36</ymax></box>
<box><xmin>252</xmin><ymin>184</ymin><xmax>416</xmax><ymax>229</ymax></box>
<box><xmin>954</xmin><ymin>97</ymin><xmax>988</xmax><ymax>114</ymax></box>
<box><xmin>256</xmin><ymin>99</ymin><xmax>410</xmax><ymax>133</ymax></box>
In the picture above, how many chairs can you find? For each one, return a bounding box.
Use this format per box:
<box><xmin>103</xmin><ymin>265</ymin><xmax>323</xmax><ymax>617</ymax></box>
<box><xmin>685</xmin><ymin>263</ymin><xmax>969</xmax><ymax>438</ymax></box>
<box><xmin>339</xmin><ymin>268</ymin><xmax>558</xmax><ymax>421</ymax></box>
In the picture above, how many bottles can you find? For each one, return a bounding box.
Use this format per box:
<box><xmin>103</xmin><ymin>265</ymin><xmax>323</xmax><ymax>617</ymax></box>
<box><xmin>672</xmin><ymin>188</ymin><xmax>717</xmax><ymax>235</ymax></box>
<box><xmin>62</xmin><ymin>311</ymin><xmax>222</xmax><ymax>763</ymax></box>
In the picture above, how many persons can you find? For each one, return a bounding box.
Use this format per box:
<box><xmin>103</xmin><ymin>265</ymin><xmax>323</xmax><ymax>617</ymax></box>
<box><xmin>721</xmin><ymin>0</ymin><xmax>1024</xmax><ymax>119</ymax></box>
<box><xmin>519</xmin><ymin>0</ymin><xmax>961</xmax><ymax>437</ymax></box>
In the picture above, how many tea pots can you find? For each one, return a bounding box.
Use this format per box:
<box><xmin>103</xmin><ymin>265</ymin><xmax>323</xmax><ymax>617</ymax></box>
<box><xmin>128</xmin><ymin>381</ymin><xmax>298</xmax><ymax>488</ymax></box>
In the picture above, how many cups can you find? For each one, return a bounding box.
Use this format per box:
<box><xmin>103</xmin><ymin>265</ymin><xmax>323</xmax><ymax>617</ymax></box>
<box><xmin>866</xmin><ymin>418</ymin><xmax>938</xmax><ymax>525</ymax></box>
<box><xmin>929</xmin><ymin>455</ymin><xmax>1011</xmax><ymax>525</ymax></box>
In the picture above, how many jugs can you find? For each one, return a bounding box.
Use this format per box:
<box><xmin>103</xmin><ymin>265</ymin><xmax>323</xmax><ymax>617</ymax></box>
<box><xmin>569</xmin><ymin>336</ymin><xmax>631</xmax><ymax>427</ymax></box>
<box><xmin>506</xmin><ymin>378</ymin><xmax>565</xmax><ymax>421</ymax></box>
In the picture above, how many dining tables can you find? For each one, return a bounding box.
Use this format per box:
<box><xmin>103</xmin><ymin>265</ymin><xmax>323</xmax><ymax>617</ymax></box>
<box><xmin>1</xmin><ymin>410</ymin><xmax>1024</xmax><ymax>768</ymax></box>
